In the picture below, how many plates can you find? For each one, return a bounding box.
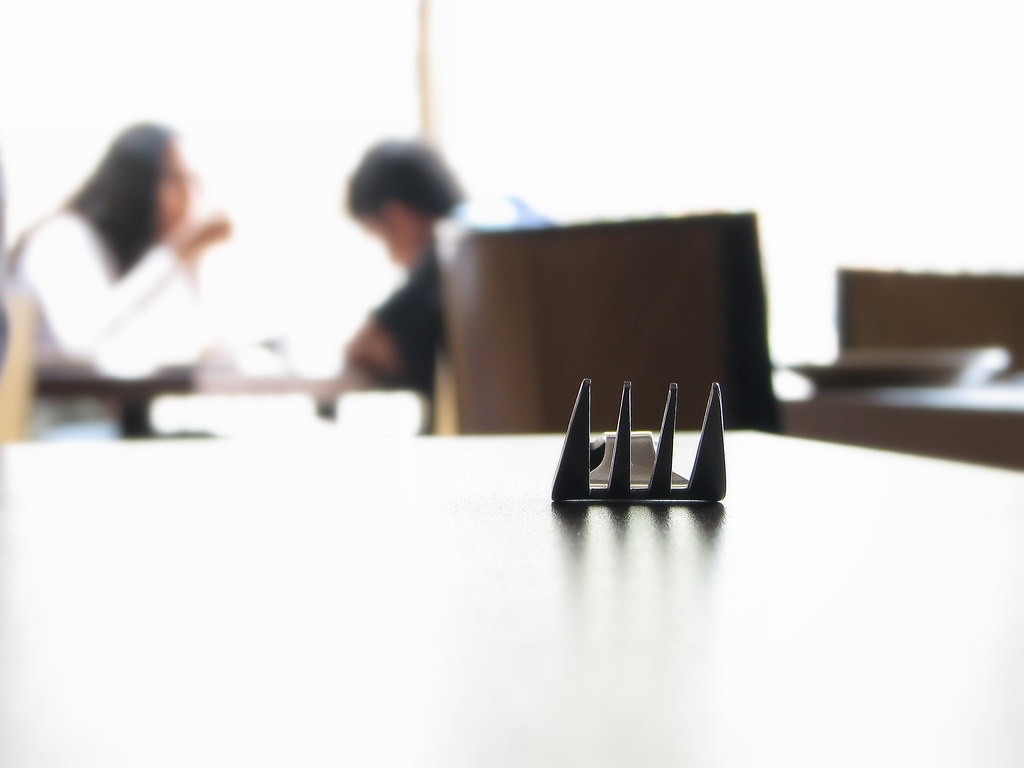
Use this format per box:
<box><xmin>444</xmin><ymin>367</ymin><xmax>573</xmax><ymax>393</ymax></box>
<box><xmin>793</xmin><ymin>352</ymin><xmax>1005</xmax><ymax>387</ymax></box>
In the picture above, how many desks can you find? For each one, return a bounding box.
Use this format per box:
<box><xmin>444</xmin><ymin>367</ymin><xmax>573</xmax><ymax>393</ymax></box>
<box><xmin>39</xmin><ymin>353</ymin><xmax>357</xmax><ymax>440</ymax></box>
<box><xmin>0</xmin><ymin>421</ymin><xmax>1020</xmax><ymax>768</ymax></box>
<box><xmin>767</xmin><ymin>371</ymin><xmax>1023</xmax><ymax>474</ymax></box>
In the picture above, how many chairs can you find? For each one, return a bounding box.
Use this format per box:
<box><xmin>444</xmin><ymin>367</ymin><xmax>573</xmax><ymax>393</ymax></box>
<box><xmin>446</xmin><ymin>206</ymin><xmax>782</xmax><ymax>443</ymax></box>
<box><xmin>832</xmin><ymin>267</ymin><xmax>1024</xmax><ymax>376</ymax></box>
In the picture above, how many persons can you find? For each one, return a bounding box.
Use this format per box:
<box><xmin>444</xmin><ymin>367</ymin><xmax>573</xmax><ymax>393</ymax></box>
<box><xmin>342</xmin><ymin>137</ymin><xmax>554</xmax><ymax>429</ymax></box>
<box><xmin>7</xmin><ymin>123</ymin><xmax>235</xmax><ymax>438</ymax></box>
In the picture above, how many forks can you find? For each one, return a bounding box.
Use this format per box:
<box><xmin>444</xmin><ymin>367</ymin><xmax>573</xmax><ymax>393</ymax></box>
<box><xmin>549</xmin><ymin>377</ymin><xmax>727</xmax><ymax>502</ymax></box>
